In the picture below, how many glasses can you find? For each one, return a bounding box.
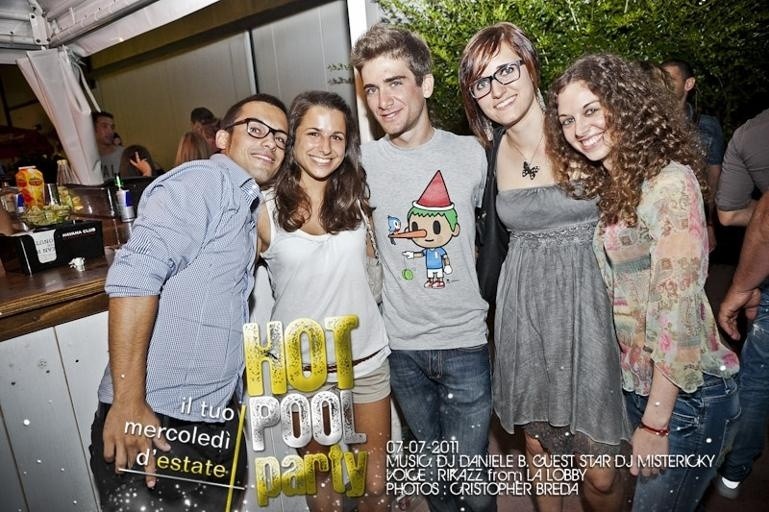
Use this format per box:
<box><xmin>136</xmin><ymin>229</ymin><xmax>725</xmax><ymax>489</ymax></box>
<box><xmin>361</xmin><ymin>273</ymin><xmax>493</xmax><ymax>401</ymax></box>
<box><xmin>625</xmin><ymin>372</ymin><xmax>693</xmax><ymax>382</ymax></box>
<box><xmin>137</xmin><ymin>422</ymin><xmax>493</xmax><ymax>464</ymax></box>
<box><xmin>468</xmin><ymin>59</ymin><xmax>526</xmax><ymax>100</ymax></box>
<box><xmin>223</xmin><ymin>117</ymin><xmax>294</xmax><ymax>154</ymax></box>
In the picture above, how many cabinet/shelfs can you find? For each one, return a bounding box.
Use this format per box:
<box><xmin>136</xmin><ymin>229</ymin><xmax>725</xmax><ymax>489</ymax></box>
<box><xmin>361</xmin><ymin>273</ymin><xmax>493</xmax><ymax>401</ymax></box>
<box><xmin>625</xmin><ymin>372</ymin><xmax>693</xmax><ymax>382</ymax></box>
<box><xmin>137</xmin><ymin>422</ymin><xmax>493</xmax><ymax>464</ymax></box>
<box><xmin>1</xmin><ymin>311</ymin><xmax>403</xmax><ymax>511</ymax></box>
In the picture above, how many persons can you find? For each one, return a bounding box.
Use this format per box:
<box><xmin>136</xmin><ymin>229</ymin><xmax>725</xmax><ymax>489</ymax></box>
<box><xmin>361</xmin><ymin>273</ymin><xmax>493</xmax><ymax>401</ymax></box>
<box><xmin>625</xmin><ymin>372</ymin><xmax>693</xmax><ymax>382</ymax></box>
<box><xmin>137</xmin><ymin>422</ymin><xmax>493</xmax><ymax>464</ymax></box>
<box><xmin>88</xmin><ymin>93</ymin><xmax>290</xmax><ymax>511</ymax></box>
<box><xmin>351</xmin><ymin>24</ymin><xmax>497</xmax><ymax>512</ymax></box>
<box><xmin>545</xmin><ymin>50</ymin><xmax>742</xmax><ymax>512</ymax></box>
<box><xmin>257</xmin><ymin>90</ymin><xmax>410</xmax><ymax>512</ymax></box>
<box><xmin>94</xmin><ymin>106</ymin><xmax>223</xmax><ymax>180</ymax></box>
<box><xmin>459</xmin><ymin>21</ymin><xmax>633</xmax><ymax>512</ymax></box>
<box><xmin>718</xmin><ymin>188</ymin><xmax>769</xmax><ymax>341</ymax></box>
<box><xmin>716</xmin><ymin>107</ymin><xmax>769</xmax><ymax>499</ymax></box>
<box><xmin>659</xmin><ymin>58</ymin><xmax>726</xmax><ymax>253</ymax></box>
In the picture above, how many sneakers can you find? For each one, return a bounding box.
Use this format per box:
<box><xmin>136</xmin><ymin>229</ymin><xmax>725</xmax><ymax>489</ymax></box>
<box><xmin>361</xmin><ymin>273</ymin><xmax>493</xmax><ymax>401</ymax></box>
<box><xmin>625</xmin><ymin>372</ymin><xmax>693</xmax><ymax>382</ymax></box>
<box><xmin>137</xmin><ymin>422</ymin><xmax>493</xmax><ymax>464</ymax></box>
<box><xmin>715</xmin><ymin>473</ymin><xmax>741</xmax><ymax>500</ymax></box>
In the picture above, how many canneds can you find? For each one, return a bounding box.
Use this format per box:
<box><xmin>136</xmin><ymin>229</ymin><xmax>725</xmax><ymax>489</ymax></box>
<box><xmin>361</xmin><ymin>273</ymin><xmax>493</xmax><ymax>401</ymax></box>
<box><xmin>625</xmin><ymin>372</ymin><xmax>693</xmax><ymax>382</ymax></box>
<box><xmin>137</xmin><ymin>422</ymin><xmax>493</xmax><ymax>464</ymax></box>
<box><xmin>13</xmin><ymin>193</ymin><xmax>26</xmax><ymax>214</ymax></box>
<box><xmin>115</xmin><ymin>189</ymin><xmax>136</xmax><ymax>223</ymax></box>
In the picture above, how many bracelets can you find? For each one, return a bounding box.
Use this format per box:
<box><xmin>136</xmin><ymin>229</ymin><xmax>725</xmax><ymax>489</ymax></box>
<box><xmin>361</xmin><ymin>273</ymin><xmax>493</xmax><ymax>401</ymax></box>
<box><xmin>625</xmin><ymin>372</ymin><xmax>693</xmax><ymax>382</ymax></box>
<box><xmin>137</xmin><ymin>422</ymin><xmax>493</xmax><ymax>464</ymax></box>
<box><xmin>638</xmin><ymin>421</ymin><xmax>670</xmax><ymax>436</ymax></box>
<box><xmin>707</xmin><ymin>223</ymin><xmax>714</xmax><ymax>228</ymax></box>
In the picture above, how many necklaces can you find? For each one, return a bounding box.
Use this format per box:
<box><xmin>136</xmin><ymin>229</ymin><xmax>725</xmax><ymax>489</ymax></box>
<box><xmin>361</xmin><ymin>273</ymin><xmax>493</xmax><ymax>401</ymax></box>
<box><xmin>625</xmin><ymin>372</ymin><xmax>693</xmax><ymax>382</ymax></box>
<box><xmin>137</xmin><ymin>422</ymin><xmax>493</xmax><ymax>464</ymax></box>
<box><xmin>512</xmin><ymin>134</ymin><xmax>543</xmax><ymax>180</ymax></box>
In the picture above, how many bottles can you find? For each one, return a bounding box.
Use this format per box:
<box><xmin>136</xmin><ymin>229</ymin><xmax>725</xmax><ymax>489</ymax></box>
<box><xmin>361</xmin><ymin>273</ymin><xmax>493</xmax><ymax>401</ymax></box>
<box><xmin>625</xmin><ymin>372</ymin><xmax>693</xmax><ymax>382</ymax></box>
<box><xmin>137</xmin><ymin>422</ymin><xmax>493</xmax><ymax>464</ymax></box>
<box><xmin>113</xmin><ymin>171</ymin><xmax>135</xmax><ymax>224</ymax></box>
<box><xmin>54</xmin><ymin>158</ymin><xmax>81</xmax><ymax>205</ymax></box>
<box><xmin>0</xmin><ymin>165</ymin><xmax>59</xmax><ymax>215</ymax></box>
